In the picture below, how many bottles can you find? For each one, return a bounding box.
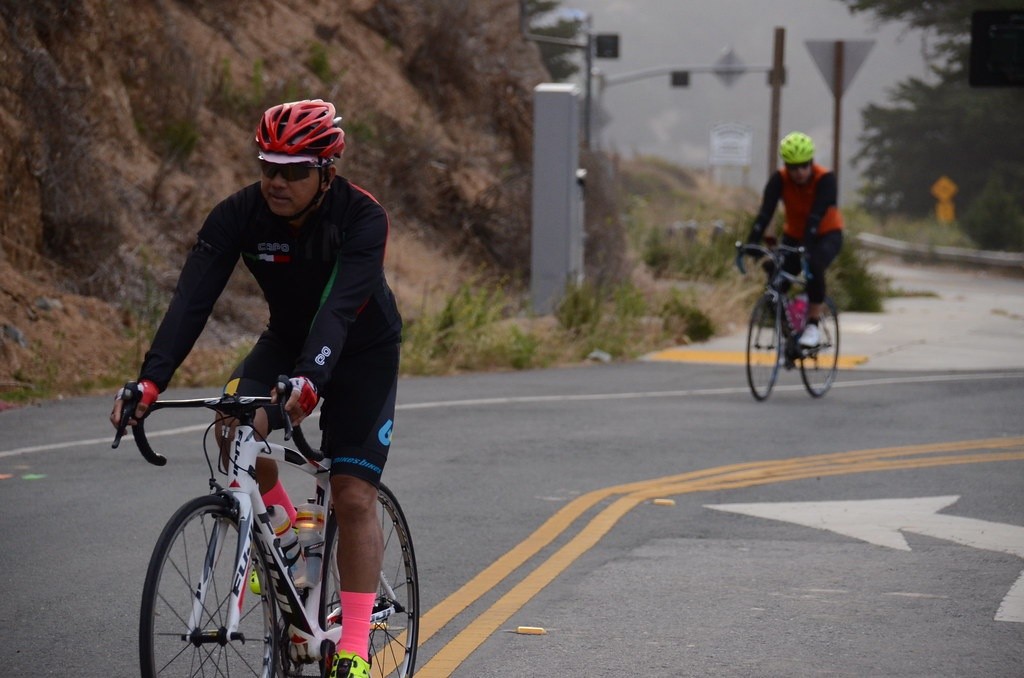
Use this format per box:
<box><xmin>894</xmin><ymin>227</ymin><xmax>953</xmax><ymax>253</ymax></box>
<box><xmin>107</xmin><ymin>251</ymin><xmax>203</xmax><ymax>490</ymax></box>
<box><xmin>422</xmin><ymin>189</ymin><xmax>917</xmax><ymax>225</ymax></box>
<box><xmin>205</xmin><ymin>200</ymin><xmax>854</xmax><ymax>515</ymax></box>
<box><xmin>295</xmin><ymin>497</ymin><xmax>325</xmax><ymax>589</ymax></box>
<box><xmin>267</xmin><ymin>503</ymin><xmax>306</xmax><ymax>580</ymax></box>
<box><xmin>792</xmin><ymin>292</ymin><xmax>808</xmax><ymax>328</ymax></box>
<box><xmin>787</xmin><ymin>297</ymin><xmax>803</xmax><ymax>332</ymax></box>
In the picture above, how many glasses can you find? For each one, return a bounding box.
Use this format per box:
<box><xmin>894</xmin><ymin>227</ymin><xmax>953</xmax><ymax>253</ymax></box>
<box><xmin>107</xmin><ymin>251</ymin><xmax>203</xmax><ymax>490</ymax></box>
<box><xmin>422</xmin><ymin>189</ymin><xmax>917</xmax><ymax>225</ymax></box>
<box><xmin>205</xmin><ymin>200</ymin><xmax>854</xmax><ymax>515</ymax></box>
<box><xmin>261</xmin><ymin>161</ymin><xmax>323</xmax><ymax>180</ymax></box>
<box><xmin>785</xmin><ymin>162</ymin><xmax>810</xmax><ymax>169</ymax></box>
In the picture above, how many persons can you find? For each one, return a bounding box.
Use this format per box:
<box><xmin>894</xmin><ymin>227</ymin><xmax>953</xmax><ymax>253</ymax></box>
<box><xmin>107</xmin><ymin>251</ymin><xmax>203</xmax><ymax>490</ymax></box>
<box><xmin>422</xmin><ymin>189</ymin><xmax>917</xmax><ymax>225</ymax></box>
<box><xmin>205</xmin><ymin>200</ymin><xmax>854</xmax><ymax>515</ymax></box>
<box><xmin>744</xmin><ymin>132</ymin><xmax>843</xmax><ymax>368</ymax></box>
<box><xmin>112</xmin><ymin>100</ymin><xmax>403</xmax><ymax>678</ymax></box>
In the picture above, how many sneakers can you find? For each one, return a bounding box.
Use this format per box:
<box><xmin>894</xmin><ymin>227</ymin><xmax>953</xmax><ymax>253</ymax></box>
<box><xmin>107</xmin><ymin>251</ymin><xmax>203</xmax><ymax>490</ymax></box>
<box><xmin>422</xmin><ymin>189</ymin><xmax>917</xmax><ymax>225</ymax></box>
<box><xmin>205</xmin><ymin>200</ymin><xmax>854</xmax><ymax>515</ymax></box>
<box><xmin>798</xmin><ymin>325</ymin><xmax>821</xmax><ymax>346</ymax></box>
<box><xmin>249</xmin><ymin>567</ymin><xmax>261</xmax><ymax>593</ymax></box>
<box><xmin>330</xmin><ymin>653</ymin><xmax>375</xmax><ymax>678</ymax></box>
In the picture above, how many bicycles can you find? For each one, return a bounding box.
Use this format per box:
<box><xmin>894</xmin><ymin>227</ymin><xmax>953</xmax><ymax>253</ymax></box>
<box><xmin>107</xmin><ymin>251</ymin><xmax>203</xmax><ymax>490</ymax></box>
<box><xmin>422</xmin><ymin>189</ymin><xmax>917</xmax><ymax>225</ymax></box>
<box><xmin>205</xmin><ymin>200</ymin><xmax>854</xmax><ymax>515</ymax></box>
<box><xmin>735</xmin><ymin>244</ymin><xmax>842</xmax><ymax>400</ymax></box>
<box><xmin>109</xmin><ymin>368</ymin><xmax>420</xmax><ymax>677</ymax></box>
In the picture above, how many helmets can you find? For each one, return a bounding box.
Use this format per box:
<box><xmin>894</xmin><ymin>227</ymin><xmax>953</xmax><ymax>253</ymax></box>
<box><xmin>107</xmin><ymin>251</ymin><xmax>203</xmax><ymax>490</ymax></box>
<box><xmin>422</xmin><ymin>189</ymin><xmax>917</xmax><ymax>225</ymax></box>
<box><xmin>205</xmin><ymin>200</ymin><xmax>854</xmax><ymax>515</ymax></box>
<box><xmin>256</xmin><ymin>99</ymin><xmax>346</xmax><ymax>157</ymax></box>
<box><xmin>780</xmin><ymin>131</ymin><xmax>816</xmax><ymax>164</ymax></box>
